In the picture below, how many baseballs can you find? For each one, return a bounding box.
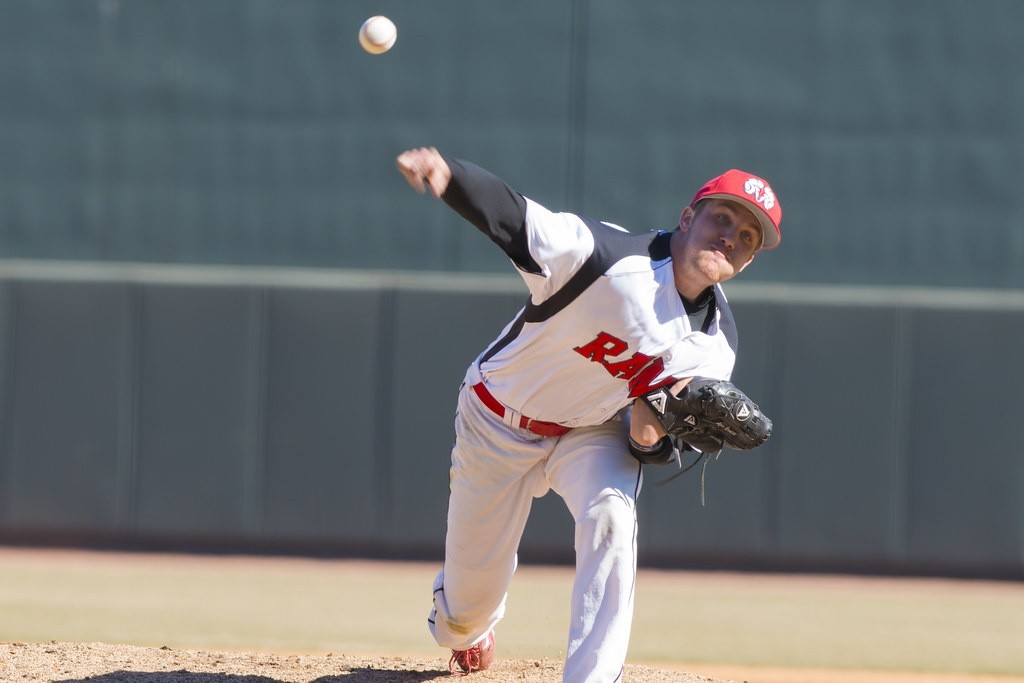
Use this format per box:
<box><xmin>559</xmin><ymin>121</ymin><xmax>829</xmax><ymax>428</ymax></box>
<box><xmin>359</xmin><ymin>14</ymin><xmax>398</xmax><ymax>55</ymax></box>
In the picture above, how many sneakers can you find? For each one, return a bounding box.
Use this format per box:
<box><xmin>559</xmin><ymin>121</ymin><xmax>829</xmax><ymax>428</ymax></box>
<box><xmin>449</xmin><ymin>629</ymin><xmax>495</xmax><ymax>676</ymax></box>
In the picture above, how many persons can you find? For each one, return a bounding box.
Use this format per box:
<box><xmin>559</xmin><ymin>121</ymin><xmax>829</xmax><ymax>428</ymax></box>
<box><xmin>394</xmin><ymin>144</ymin><xmax>782</xmax><ymax>683</ymax></box>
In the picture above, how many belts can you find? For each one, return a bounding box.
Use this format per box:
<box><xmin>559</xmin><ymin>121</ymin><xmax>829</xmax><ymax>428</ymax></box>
<box><xmin>472</xmin><ymin>382</ymin><xmax>572</xmax><ymax>437</ymax></box>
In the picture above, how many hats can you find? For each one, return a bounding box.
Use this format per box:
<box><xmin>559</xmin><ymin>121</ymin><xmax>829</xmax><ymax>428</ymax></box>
<box><xmin>691</xmin><ymin>168</ymin><xmax>782</xmax><ymax>249</ymax></box>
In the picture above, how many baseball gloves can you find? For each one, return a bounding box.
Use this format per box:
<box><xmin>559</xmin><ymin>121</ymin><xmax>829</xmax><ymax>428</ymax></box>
<box><xmin>641</xmin><ymin>376</ymin><xmax>774</xmax><ymax>454</ymax></box>
